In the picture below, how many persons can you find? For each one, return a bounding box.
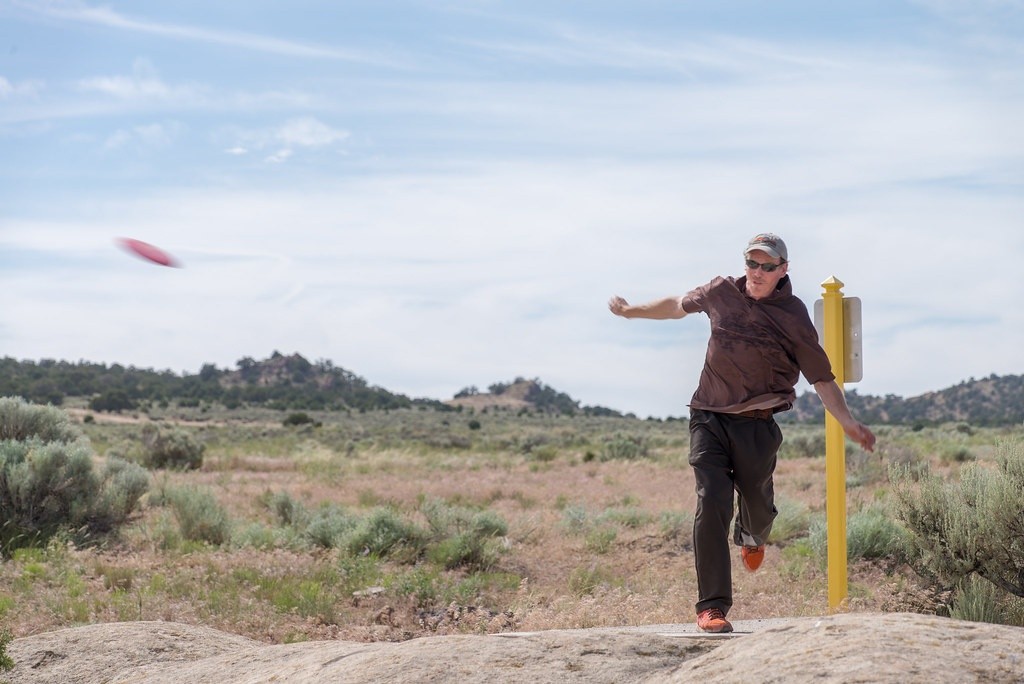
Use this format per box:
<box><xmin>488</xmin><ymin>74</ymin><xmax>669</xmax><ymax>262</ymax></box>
<box><xmin>609</xmin><ymin>233</ymin><xmax>875</xmax><ymax>633</ymax></box>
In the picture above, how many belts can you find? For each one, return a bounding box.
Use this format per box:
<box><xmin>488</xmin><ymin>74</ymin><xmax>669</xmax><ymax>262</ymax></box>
<box><xmin>738</xmin><ymin>410</ymin><xmax>768</xmax><ymax>420</ymax></box>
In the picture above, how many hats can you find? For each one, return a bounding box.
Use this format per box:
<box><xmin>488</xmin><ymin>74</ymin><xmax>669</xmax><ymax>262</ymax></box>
<box><xmin>743</xmin><ymin>232</ymin><xmax>787</xmax><ymax>261</ymax></box>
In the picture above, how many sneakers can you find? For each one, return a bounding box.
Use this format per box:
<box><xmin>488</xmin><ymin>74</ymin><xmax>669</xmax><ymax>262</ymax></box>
<box><xmin>696</xmin><ymin>607</ymin><xmax>733</xmax><ymax>633</ymax></box>
<box><xmin>742</xmin><ymin>542</ymin><xmax>764</xmax><ymax>571</ymax></box>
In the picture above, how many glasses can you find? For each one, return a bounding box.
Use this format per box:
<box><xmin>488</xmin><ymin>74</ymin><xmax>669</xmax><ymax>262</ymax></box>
<box><xmin>746</xmin><ymin>258</ymin><xmax>787</xmax><ymax>272</ymax></box>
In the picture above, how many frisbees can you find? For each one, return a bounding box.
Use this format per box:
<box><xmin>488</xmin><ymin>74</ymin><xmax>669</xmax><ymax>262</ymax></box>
<box><xmin>119</xmin><ymin>236</ymin><xmax>173</xmax><ymax>266</ymax></box>
